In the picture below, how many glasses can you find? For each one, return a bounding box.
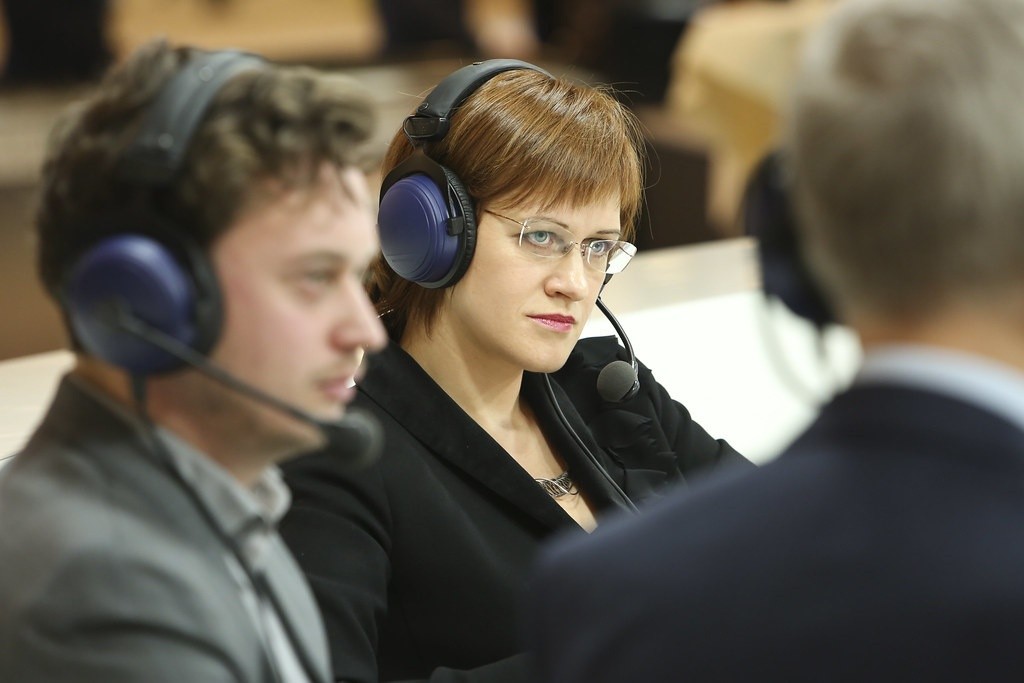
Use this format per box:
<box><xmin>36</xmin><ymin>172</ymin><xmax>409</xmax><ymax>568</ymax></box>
<box><xmin>484</xmin><ymin>208</ymin><xmax>637</xmax><ymax>274</ymax></box>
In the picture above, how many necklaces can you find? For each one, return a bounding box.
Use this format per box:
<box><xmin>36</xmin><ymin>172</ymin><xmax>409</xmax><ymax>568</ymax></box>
<box><xmin>537</xmin><ymin>471</ymin><xmax>573</xmax><ymax>499</ymax></box>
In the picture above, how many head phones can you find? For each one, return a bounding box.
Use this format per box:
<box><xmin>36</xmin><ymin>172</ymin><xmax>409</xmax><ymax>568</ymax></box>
<box><xmin>378</xmin><ymin>58</ymin><xmax>613</xmax><ymax>289</ymax></box>
<box><xmin>734</xmin><ymin>147</ymin><xmax>848</xmax><ymax>330</ymax></box>
<box><xmin>53</xmin><ymin>50</ymin><xmax>275</xmax><ymax>385</ymax></box>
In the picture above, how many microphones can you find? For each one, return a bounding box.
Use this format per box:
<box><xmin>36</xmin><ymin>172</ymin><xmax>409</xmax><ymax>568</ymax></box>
<box><xmin>594</xmin><ymin>297</ymin><xmax>641</xmax><ymax>404</ymax></box>
<box><xmin>122</xmin><ymin>316</ymin><xmax>383</xmax><ymax>464</ymax></box>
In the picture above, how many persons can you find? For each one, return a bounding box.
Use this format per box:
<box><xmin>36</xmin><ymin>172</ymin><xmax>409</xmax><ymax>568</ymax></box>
<box><xmin>0</xmin><ymin>40</ymin><xmax>390</xmax><ymax>683</ymax></box>
<box><xmin>276</xmin><ymin>58</ymin><xmax>760</xmax><ymax>683</ymax></box>
<box><xmin>515</xmin><ymin>11</ymin><xmax>1024</xmax><ymax>683</ymax></box>
